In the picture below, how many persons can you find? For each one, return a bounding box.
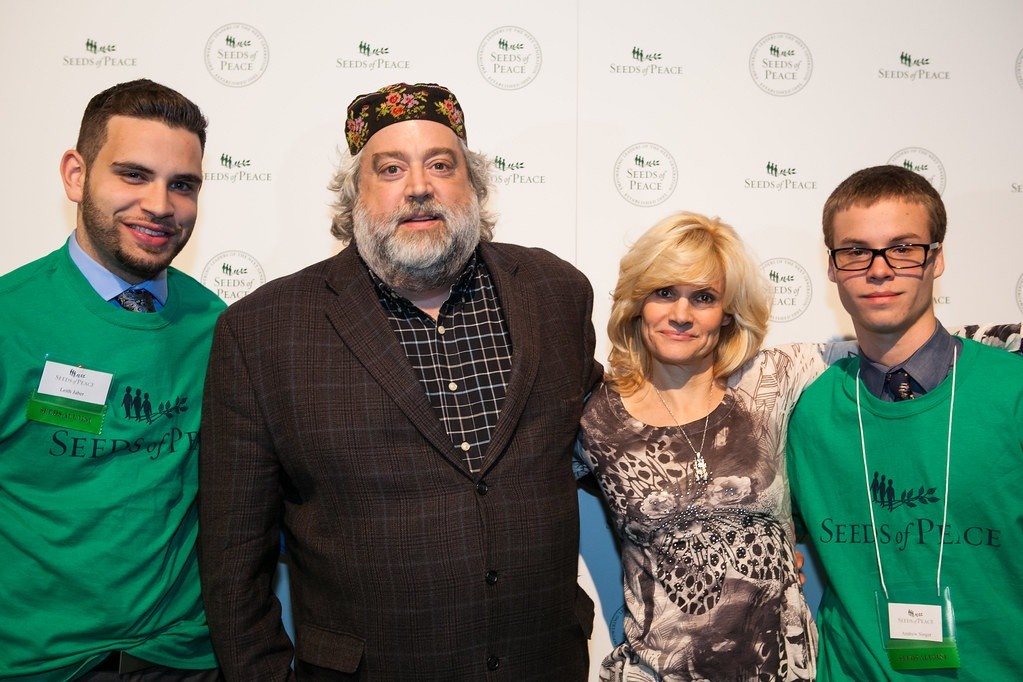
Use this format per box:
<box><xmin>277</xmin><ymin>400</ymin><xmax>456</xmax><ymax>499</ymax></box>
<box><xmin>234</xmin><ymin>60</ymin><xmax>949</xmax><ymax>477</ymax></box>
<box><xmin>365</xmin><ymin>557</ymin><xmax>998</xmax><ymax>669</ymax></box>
<box><xmin>784</xmin><ymin>165</ymin><xmax>1022</xmax><ymax>682</ymax></box>
<box><xmin>0</xmin><ymin>79</ymin><xmax>227</xmax><ymax>682</ymax></box>
<box><xmin>198</xmin><ymin>81</ymin><xmax>807</xmax><ymax>682</ymax></box>
<box><xmin>572</xmin><ymin>211</ymin><xmax>1023</xmax><ymax>682</ymax></box>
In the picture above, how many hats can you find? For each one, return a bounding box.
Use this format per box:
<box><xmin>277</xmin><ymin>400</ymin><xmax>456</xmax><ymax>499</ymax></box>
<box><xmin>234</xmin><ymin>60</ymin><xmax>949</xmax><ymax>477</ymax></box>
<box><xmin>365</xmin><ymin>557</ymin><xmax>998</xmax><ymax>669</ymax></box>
<box><xmin>345</xmin><ymin>82</ymin><xmax>468</xmax><ymax>155</ymax></box>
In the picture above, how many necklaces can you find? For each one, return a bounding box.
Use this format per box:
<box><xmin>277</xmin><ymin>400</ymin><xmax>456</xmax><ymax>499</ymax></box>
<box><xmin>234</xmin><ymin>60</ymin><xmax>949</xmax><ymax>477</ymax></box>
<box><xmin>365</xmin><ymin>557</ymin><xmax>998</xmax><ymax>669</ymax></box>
<box><xmin>651</xmin><ymin>377</ymin><xmax>714</xmax><ymax>482</ymax></box>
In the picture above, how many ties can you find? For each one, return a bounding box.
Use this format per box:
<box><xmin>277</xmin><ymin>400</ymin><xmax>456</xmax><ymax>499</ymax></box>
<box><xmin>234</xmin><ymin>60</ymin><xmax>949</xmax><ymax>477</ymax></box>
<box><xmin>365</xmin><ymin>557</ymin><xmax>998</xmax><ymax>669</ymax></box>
<box><xmin>886</xmin><ymin>370</ymin><xmax>916</xmax><ymax>402</ymax></box>
<box><xmin>116</xmin><ymin>288</ymin><xmax>156</xmax><ymax>313</ymax></box>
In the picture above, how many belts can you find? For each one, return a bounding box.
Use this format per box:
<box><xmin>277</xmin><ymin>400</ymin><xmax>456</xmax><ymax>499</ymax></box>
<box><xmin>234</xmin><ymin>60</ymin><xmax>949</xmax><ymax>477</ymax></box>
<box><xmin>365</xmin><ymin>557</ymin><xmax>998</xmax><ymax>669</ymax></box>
<box><xmin>90</xmin><ymin>650</ymin><xmax>152</xmax><ymax>675</ymax></box>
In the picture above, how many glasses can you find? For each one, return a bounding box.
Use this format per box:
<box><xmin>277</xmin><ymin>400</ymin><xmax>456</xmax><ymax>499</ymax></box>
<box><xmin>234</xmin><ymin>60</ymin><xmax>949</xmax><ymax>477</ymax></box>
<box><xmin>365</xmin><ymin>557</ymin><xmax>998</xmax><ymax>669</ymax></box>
<box><xmin>829</xmin><ymin>241</ymin><xmax>939</xmax><ymax>272</ymax></box>
